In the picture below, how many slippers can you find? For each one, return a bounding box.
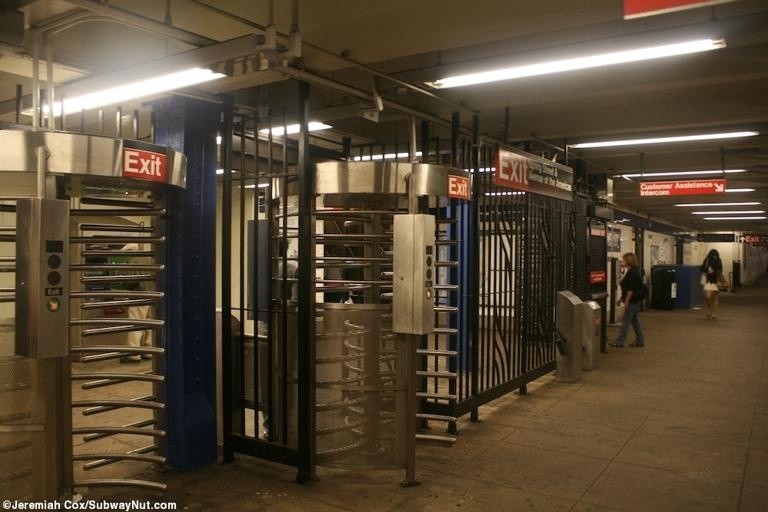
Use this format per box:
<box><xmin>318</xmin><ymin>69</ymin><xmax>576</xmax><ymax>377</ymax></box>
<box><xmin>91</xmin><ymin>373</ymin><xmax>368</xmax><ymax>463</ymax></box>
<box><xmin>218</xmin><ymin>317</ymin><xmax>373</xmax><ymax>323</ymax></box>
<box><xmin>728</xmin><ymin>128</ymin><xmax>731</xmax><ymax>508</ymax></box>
<box><xmin>629</xmin><ymin>340</ymin><xmax>644</xmax><ymax>347</ymax></box>
<box><xmin>706</xmin><ymin>313</ymin><xmax>716</xmax><ymax>319</ymax></box>
<box><xmin>609</xmin><ymin>342</ymin><xmax>624</xmax><ymax>347</ymax></box>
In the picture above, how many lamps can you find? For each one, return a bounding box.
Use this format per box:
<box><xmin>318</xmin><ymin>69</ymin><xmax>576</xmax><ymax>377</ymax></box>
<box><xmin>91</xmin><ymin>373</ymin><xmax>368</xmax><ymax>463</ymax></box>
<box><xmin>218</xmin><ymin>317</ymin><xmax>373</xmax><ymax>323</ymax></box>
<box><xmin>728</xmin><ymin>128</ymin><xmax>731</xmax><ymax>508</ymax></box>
<box><xmin>260</xmin><ymin>103</ymin><xmax>379</xmax><ymax>139</ymax></box>
<box><xmin>21</xmin><ymin>34</ymin><xmax>283</xmax><ymax>118</ymax></box>
<box><xmin>352</xmin><ymin>131</ymin><xmax>766</xmax><ymax>222</ymax></box>
<box><xmin>425</xmin><ymin>36</ymin><xmax>727</xmax><ymax>91</ymax></box>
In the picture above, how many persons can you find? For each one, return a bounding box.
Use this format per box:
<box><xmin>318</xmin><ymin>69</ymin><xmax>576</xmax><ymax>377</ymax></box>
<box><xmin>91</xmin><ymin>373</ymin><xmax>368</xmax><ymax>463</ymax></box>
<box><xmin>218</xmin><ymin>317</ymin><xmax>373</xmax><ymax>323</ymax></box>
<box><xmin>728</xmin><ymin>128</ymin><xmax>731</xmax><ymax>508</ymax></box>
<box><xmin>324</xmin><ymin>248</ymin><xmax>344</xmax><ymax>303</ymax></box>
<box><xmin>118</xmin><ymin>242</ymin><xmax>155</xmax><ymax>364</ymax></box>
<box><xmin>700</xmin><ymin>249</ymin><xmax>723</xmax><ymax>320</ymax></box>
<box><xmin>606</xmin><ymin>252</ymin><xmax>646</xmax><ymax>348</ymax></box>
<box><xmin>261</xmin><ymin>232</ymin><xmax>298</xmax><ymax>440</ymax></box>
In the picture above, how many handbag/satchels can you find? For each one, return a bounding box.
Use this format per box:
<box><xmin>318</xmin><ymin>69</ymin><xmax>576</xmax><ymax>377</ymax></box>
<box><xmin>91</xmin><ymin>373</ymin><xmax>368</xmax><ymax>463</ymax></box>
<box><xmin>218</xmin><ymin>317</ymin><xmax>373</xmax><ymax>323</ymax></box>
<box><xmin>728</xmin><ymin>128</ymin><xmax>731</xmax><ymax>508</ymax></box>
<box><xmin>632</xmin><ymin>285</ymin><xmax>649</xmax><ymax>305</ymax></box>
<box><xmin>699</xmin><ymin>272</ymin><xmax>707</xmax><ymax>286</ymax></box>
<box><xmin>716</xmin><ymin>281</ymin><xmax>730</xmax><ymax>292</ymax></box>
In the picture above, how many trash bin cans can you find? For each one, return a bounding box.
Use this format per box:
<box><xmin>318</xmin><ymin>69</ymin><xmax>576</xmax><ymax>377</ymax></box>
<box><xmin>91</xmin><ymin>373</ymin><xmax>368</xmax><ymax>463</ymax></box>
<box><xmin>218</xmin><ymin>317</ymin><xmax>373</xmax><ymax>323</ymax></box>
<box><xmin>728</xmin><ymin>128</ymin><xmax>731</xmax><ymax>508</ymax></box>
<box><xmin>651</xmin><ymin>266</ymin><xmax>703</xmax><ymax>310</ymax></box>
